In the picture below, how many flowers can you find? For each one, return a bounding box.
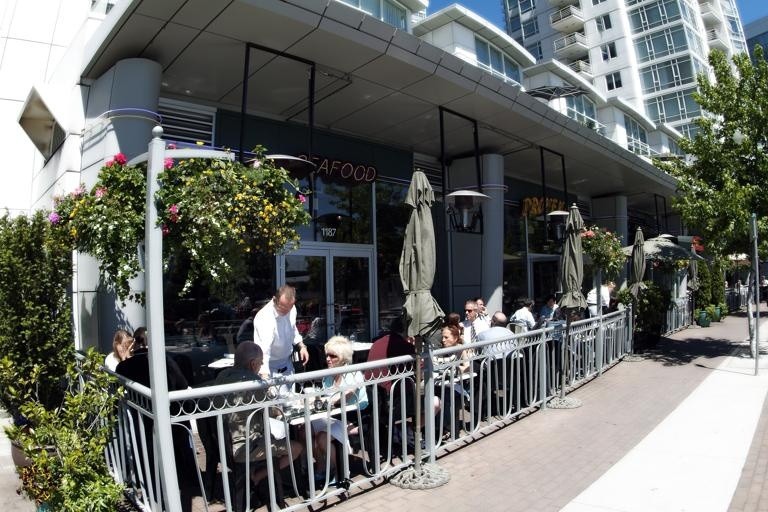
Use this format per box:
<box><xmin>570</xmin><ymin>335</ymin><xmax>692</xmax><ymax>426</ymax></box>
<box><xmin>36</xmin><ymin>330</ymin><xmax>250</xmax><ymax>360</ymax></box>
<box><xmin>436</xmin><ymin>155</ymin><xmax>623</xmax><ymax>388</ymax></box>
<box><xmin>49</xmin><ymin>142</ymin><xmax>308</xmax><ymax>311</ymax></box>
<box><xmin>581</xmin><ymin>226</ymin><xmax>629</xmax><ymax>278</ymax></box>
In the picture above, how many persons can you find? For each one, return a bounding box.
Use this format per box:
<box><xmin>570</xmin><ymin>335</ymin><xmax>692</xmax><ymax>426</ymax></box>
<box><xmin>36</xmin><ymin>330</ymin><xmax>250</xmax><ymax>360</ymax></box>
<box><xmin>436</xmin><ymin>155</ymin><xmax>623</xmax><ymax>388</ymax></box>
<box><xmin>295</xmin><ymin>299</ymin><xmax>369</xmax><ymax>370</ymax></box>
<box><xmin>761</xmin><ymin>275</ymin><xmax>768</xmax><ymax>306</ymax></box>
<box><xmin>254</xmin><ymin>286</ymin><xmax>310</xmax><ymax>392</ymax></box>
<box><xmin>448</xmin><ymin>296</ymin><xmax>566</xmax><ymax>408</ymax></box>
<box><xmin>304</xmin><ymin>336</ymin><xmax>369</xmax><ymax>486</ymax></box>
<box><xmin>365</xmin><ymin>319</ymin><xmax>441</xmax><ymax>448</ymax></box>
<box><xmin>441</xmin><ymin>325</ymin><xmax>469</xmax><ymax>407</ymax></box>
<box><xmin>587</xmin><ymin>281</ymin><xmax>616</xmax><ymax>325</ymax></box>
<box><xmin>166</xmin><ymin>293</ymin><xmax>260</xmax><ymax>383</ymax></box>
<box><xmin>105</xmin><ymin>332</ymin><xmax>135</xmax><ymax>373</ymax></box>
<box><xmin>115</xmin><ymin>328</ymin><xmax>188</xmax><ymax>417</ymax></box>
<box><xmin>215</xmin><ymin>340</ymin><xmax>302</xmax><ymax>512</ymax></box>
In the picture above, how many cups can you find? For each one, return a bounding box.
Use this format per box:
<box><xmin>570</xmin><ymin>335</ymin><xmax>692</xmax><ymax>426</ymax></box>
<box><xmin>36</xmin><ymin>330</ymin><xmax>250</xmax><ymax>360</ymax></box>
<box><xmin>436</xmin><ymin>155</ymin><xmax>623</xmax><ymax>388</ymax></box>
<box><xmin>303</xmin><ymin>387</ymin><xmax>316</xmax><ymax>410</ymax></box>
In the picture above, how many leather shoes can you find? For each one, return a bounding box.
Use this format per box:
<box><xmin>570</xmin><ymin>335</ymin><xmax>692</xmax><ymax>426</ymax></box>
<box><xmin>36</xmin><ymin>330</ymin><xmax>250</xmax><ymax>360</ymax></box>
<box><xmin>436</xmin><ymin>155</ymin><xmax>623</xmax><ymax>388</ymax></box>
<box><xmin>441</xmin><ymin>427</ymin><xmax>451</xmax><ymax>440</ymax></box>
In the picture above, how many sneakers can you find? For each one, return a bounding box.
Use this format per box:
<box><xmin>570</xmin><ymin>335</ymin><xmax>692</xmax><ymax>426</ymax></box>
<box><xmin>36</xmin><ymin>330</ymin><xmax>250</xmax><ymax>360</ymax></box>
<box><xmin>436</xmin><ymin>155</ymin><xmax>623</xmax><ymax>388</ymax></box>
<box><xmin>398</xmin><ymin>423</ymin><xmax>424</xmax><ymax>449</ymax></box>
<box><xmin>245</xmin><ymin>472</ymin><xmax>262</xmax><ymax>507</ymax></box>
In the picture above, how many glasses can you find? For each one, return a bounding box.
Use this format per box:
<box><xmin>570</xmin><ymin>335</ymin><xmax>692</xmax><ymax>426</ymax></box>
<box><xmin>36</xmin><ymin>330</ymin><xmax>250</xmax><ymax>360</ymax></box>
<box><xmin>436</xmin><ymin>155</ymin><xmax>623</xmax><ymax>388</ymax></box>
<box><xmin>326</xmin><ymin>354</ymin><xmax>337</xmax><ymax>358</ymax></box>
<box><xmin>464</xmin><ymin>309</ymin><xmax>472</xmax><ymax>313</ymax></box>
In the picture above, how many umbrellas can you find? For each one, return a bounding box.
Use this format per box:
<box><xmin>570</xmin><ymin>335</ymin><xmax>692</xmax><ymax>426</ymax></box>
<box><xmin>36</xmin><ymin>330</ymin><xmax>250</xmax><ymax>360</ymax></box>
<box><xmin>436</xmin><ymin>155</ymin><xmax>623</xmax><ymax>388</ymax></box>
<box><xmin>396</xmin><ymin>165</ymin><xmax>447</xmax><ymax>470</ymax></box>
<box><xmin>553</xmin><ymin>202</ymin><xmax>588</xmax><ymax>401</ymax></box>
<box><xmin>618</xmin><ymin>231</ymin><xmax>706</xmax><ymax>283</ymax></box>
<box><xmin>626</xmin><ymin>226</ymin><xmax>646</xmax><ymax>355</ymax></box>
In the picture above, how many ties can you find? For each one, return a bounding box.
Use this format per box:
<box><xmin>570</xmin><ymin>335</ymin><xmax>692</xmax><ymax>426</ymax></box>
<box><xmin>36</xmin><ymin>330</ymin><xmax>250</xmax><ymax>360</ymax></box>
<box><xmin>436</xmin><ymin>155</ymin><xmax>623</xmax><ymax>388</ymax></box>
<box><xmin>471</xmin><ymin>322</ymin><xmax>478</xmax><ymax>342</ymax></box>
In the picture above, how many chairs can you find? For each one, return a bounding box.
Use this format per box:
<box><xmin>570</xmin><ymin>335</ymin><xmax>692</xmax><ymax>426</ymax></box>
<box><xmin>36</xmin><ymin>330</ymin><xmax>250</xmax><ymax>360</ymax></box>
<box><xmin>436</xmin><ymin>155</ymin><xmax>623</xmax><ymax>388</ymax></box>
<box><xmin>207</xmin><ymin>380</ymin><xmax>383</xmax><ymax>511</ymax></box>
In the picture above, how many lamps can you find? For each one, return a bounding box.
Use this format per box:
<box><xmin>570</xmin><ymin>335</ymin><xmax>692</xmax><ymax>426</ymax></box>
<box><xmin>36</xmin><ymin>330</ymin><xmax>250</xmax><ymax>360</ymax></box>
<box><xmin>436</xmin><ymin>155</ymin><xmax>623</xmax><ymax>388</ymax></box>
<box><xmin>444</xmin><ymin>189</ymin><xmax>493</xmax><ymax>232</ymax></box>
<box><xmin>548</xmin><ymin>210</ymin><xmax>570</xmax><ymax>240</ymax></box>
